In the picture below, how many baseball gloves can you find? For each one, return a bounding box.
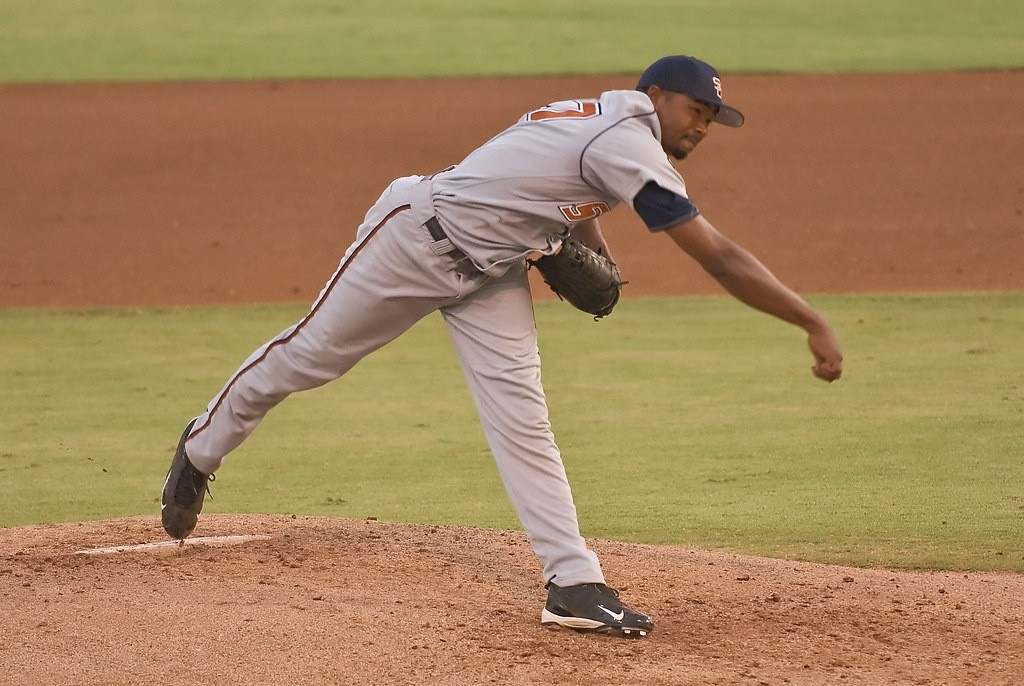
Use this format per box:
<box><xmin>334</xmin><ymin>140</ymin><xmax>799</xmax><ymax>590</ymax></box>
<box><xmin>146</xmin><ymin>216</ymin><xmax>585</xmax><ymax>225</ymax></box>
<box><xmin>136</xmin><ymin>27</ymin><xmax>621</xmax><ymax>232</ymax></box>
<box><xmin>527</xmin><ymin>234</ymin><xmax>629</xmax><ymax>322</ymax></box>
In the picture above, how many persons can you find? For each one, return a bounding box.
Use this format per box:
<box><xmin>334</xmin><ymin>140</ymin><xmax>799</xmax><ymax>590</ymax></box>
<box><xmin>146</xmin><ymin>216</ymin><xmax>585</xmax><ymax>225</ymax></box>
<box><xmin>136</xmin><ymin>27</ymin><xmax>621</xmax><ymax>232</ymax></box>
<box><xmin>161</xmin><ymin>55</ymin><xmax>844</xmax><ymax>636</ymax></box>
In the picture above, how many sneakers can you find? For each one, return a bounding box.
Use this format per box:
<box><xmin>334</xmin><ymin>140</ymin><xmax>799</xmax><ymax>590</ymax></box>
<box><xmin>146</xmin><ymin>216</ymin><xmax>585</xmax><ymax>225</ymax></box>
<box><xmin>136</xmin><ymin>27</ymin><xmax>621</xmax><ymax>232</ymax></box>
<box><xmin>161</xmin><ymin>417</ymin><xmax>215</xmax><ymax>540</ymax></box>
<box><xmin>542</xmin><ymin>581</ymin><xmax>653</xmax><ymax>637</ymax></box>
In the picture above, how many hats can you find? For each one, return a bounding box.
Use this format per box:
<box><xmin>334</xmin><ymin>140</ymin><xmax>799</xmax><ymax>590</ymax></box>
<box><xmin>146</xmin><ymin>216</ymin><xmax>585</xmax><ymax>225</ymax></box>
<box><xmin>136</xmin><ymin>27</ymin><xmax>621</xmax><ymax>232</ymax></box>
<box><xmin>637</xmin><ymin>55</ymin><xmax>744</xmax><ymax>128</ymax></box>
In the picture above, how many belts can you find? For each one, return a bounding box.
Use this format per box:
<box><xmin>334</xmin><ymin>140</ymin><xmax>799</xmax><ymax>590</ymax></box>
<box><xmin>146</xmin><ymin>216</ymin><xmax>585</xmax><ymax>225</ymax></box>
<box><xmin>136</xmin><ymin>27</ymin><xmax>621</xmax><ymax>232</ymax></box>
<box><xmin>420</xmin><ymin>167</ymin><xmax>484</xmax><ymax>278</ymax></box>
